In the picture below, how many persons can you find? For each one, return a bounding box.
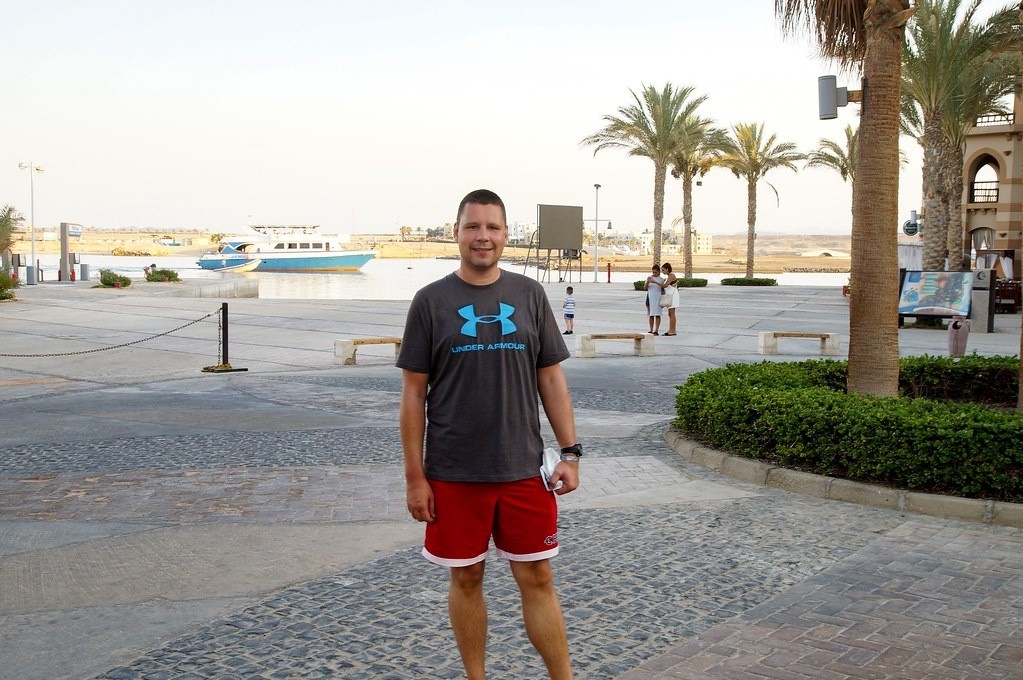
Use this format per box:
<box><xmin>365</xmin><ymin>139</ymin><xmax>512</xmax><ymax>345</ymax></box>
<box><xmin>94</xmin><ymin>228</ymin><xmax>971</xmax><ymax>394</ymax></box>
<box><xmin>562</xmin><ymin>286</ymin><xmax>576</xmax><ymax>335</ymax></box>
<box><xmin>263</xmin><ymin>230</ymin><xmax>294</xmax><ymax>234</ymax></box>
<box><xmin>394</xmin><ymin>189</ymin><xmax>584</xmax><ymax>680</ymax></box>
<box><xmin>644</xmin><ymin>263</ymin><xmax>680</xmax><ymax>336</ymax></box>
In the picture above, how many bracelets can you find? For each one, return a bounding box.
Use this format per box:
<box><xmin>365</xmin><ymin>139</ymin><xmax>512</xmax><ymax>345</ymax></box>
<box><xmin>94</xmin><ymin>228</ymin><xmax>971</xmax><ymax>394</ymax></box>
<box><xmin>561</xmin><ymin>442</ymin><xmax>583</xmax><ymax>457</ymax></box>
<box><xmin>559</xmin><ymin>455</ymin><xmax>579</xmax><ymax>462</ymax></box>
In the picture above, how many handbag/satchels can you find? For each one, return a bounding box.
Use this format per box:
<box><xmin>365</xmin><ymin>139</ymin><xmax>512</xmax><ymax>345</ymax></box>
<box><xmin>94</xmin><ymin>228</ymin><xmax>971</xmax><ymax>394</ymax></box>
<box><xmin>660</xmin><ymin>295</ymin><xmax>672</xmax><ymax>307</ymax></box>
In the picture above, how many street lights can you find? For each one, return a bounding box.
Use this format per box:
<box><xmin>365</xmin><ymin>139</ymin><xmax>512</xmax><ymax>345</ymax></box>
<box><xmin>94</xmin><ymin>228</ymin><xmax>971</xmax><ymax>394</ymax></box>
<box><xmin>592</xmin><ymin>184</ymin><xmax>601</xmax><ymax>284</ymax></box>
<box><xmin>18</xmin><ymin>160</ymin><xmax>46</xmax><ymax>286</ymax></box>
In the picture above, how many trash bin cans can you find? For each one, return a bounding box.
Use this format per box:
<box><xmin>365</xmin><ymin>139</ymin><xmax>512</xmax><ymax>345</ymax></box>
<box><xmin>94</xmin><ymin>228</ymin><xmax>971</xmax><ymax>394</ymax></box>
<box><xmin>947</xmin><ymin>316</ymin><xmax>971</xmax><ymax>357</ymax></box>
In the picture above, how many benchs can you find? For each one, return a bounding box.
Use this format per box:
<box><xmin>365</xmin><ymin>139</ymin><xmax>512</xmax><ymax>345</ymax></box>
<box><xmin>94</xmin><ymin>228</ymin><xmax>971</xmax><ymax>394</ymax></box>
<box><xmin>333</xmin><ymin>337</ymin><xmax>403</xmax><ymax>365</ymax></box>
<box><xmin>758</xmin><ymin>331</ymin><xmax>839</xmax><ymax>356</ymax></box>
<box><xmin>574</xmin><ymin>332</ymin><xmax>657</xmax><ymax>358</ymax></box>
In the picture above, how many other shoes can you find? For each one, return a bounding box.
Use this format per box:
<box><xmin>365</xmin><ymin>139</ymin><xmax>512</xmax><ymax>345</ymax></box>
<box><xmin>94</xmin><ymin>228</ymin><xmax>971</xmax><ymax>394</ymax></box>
<box><xmin>570</xmin><ymin>331</ymin><xmax>572</xmax><ymax>334</ymax></box>
<box><xmin>648</xmin><ymin>332</ymin><xmax>652</xmax><ymax>333</ymax></box>
<box><xmin>563</xmin><ymin>331</ymin><xmax>570</xmax><ymax>334</ymax></box>
<box><xmin>653</xmin><ymin>332</ymin><xmax>659</xmax><ymax>335</ymax></box>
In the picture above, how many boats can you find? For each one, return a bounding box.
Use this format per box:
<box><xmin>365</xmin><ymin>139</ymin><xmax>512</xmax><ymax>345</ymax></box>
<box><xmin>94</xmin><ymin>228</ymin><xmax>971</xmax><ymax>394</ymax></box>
<box><xmin>213</xmin><ymin>254</ymin><xmax>263</xmax><ymax>274</ymax></box>
<box><xmin>195</xmin><ymin>224</ymin><xmax>380</xmax><ymax>272</ymax></box>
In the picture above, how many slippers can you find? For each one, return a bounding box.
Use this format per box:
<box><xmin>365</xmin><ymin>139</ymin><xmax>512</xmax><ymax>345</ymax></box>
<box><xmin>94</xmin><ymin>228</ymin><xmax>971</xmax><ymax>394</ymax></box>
<box><xmin>673</xmin><ymin>334</ymin><xmax>677</xmax><ymax>335</ymax></box>
<box><xmin>662</xmin><ymin>333</ymin><xmax>673</xmax><ymax>336</ymax></box>
